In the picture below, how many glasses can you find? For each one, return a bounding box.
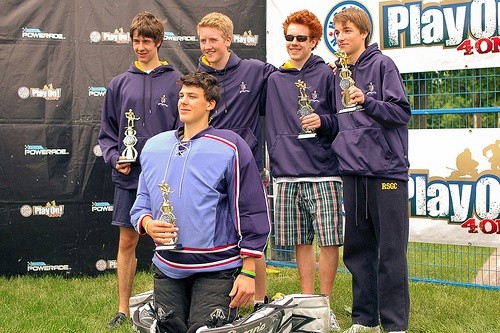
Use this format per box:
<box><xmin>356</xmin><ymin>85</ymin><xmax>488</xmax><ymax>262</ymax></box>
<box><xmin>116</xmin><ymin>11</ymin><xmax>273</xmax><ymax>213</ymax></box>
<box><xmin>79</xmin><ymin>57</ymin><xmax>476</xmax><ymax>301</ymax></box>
<box><xmin>285</xmin><ymin>35</ymin><xmax>310</xmax><ymax>42</ymax></box>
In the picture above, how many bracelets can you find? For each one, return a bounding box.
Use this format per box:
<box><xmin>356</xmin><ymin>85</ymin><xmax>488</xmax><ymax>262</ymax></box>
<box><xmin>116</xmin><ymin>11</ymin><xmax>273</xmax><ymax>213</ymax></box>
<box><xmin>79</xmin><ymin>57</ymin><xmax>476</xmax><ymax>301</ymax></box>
<box><xmin>240</xmin><ymin>269</ymin><xmax>255</xmax><ymax>279</ymax></box>
<box><xmin>145</xmin><ymin>218</ymin><xmax>151</xmax><ymax>234</ymax></box>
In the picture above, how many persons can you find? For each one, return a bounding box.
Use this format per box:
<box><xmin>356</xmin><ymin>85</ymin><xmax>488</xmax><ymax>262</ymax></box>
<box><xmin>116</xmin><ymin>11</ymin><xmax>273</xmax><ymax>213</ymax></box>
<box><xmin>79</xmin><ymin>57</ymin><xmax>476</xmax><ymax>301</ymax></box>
<box><xmin>331</xmin><ymin>8</ymin><xmax>412</xmax><ymax>333</ymax></box>
<box><xmin>172</xmin><ymin>12</ymin><xmax>338</xmax><ymax>311</ymax></box>
<box><xmin>130</xmin><ymin>69</ymin><xmax>273</xmax><ymax>333</ymax></box>
<box><xmin>98</xmin><ymin>11</ymin><xmax>185</xmax><ymax>329</ymax></box>
<box><xmin>259</xmin><ymin>10</ymin><xmax>344</xmax><ymax>331</ymax></box>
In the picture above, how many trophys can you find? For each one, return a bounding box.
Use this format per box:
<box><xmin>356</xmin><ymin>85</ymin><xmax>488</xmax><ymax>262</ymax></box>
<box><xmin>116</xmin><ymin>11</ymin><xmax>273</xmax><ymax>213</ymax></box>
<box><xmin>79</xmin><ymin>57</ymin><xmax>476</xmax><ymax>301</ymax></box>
<box><xmin>152</xmin><ymin>182</ymin><xmax>184</xmax><ymax>250</ymax></box>
<box><xmin>293</xmin><ymin>80</ymin><xmax>317</xmax><ymax>139</ymax></box>
<box><xmin>116</xmin><ymin>109</ymin><xmax>142</xmax><ymax>163</ymax></box>
<box><xmin>334</xmin><ymin>50</ymin><xmax>364</xmax><ymax>113</ymax></box>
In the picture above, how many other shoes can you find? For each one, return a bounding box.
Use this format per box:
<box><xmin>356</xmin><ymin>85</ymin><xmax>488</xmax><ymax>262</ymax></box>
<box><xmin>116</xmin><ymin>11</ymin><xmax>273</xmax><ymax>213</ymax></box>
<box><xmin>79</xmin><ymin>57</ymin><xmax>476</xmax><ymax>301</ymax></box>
<box><xmin>342</xmin><ymin>324</ymin><xmax>381</xmax><ymax>333</ymax></box>
<box><xmin>105</xmin><ymin>312</ymin><xmax>127</xmax><ymax>329</ymax></box>
